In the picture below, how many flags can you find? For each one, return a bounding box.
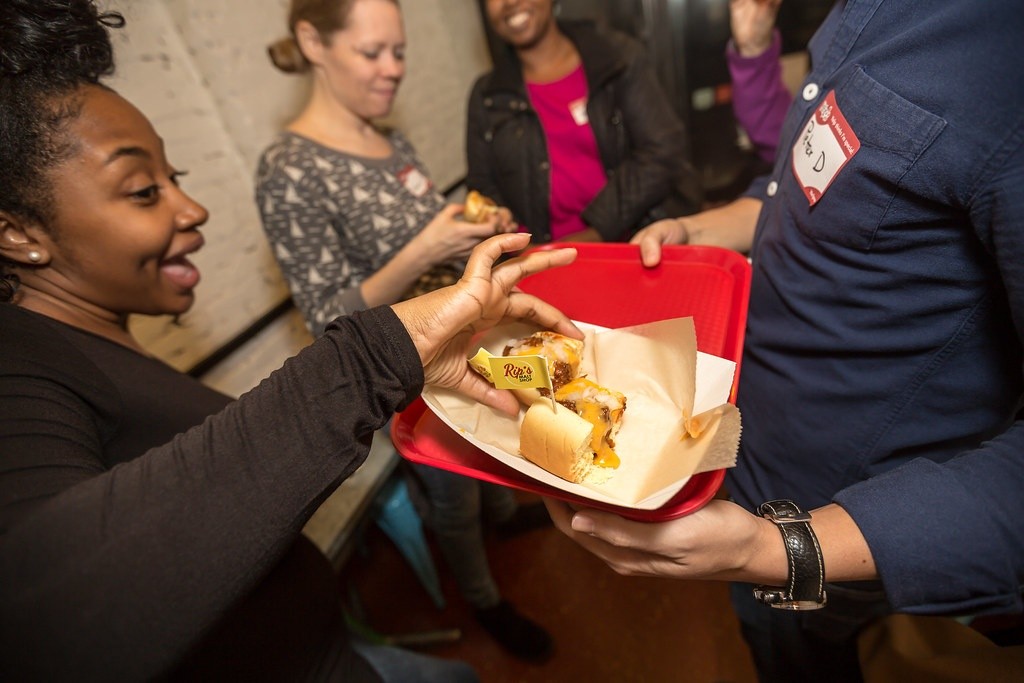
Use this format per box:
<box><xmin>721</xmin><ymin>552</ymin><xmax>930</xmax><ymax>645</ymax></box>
<box><xmin>467</xmin><ymin>347</ymin><xmax>495</xmax><ymax>383</ymax></box>
<box><xmin>488</xmin><ymin>356</ymin><xmax>553</xmax><ymax>390</ymax></box>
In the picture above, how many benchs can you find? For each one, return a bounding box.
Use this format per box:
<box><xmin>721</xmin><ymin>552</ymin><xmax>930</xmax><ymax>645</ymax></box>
<box><xmin>204</xmin><ymin>312</ymin><xmax>449</xmax><ymax>613</ymax></box>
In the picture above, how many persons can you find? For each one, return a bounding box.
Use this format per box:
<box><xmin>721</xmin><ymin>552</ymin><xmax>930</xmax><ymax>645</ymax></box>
<box><xmin>726</xmin><ymin>0</ymin><xmax>794</xmax><ymax>167</ymax></box>
<box><xmin>541</xmin><ymin>0</ymin><xmax>1024</xmax><ymax>683</ymax></box>
<box><xmin>469</xmin><ymin>0</ymin><xmax>686</xmax><ymax>258</ymax></box>
<box><xmin>256</xmin><ymin>0</ymin><xmax>556</xmax><ymax>663</ymax></box>
<box><xmin>0</xmin><ymin>0</ymin><xmax>585</xmax><ymax>683</ymax></box>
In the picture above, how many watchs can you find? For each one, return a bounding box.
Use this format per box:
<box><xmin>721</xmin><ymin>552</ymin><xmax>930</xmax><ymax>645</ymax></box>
<box><xmin>752</xmin><ymin>499</ymin><xmax>827</xmax><ymax>611</ymax></box>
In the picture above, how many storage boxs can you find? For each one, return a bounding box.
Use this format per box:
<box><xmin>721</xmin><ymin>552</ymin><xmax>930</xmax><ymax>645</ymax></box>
<box><xmin>421</xmin><ymin>319</ymin><xmax>737</xmax><ymax>511</ymax></box>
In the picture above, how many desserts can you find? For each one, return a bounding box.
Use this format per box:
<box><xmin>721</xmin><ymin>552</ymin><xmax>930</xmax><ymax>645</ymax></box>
<box><xmin>505</xmin><ymin>330</ymin><xmax>627</xmax><ymax>484</ymax></box>
<box><xmin>463</xmin><ymin>190</ymin><xmax>498</xmax><ymax>221</ymax></box>
